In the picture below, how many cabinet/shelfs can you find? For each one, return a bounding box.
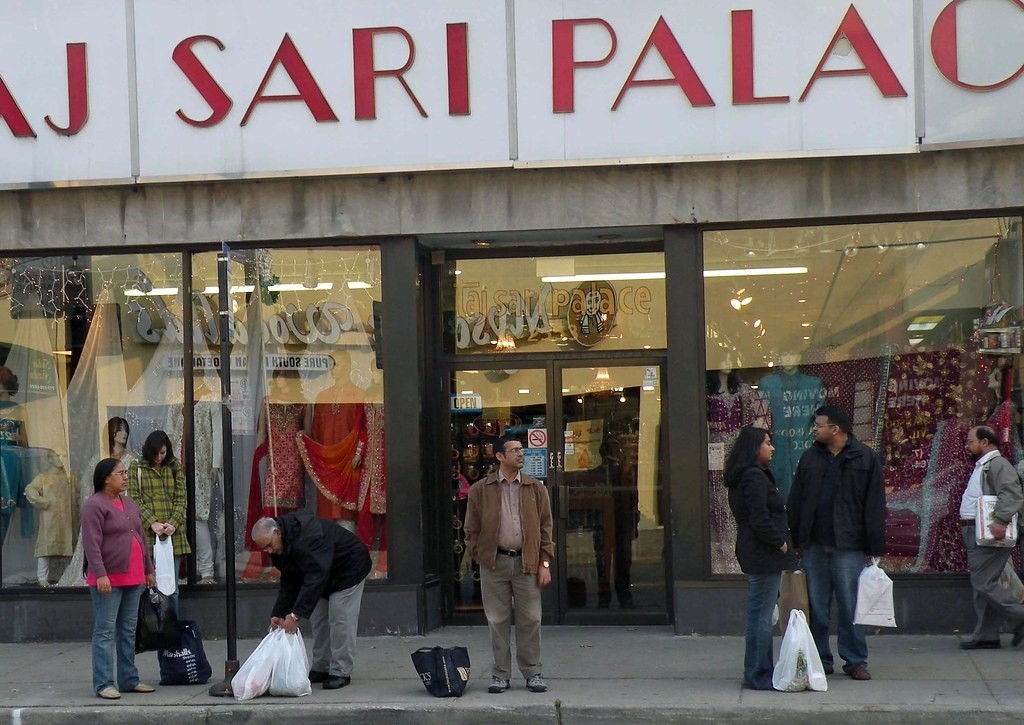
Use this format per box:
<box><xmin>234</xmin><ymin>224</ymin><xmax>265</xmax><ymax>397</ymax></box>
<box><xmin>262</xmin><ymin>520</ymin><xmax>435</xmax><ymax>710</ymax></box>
<box><xmin>975</xmin><ymin>326</ymin><xmax>1022</xmax><ymax>355</ymax></box>
<box><xmin>450</xmin><ymin>435</ymin><xmax>499</xmax><ymax>464</ymax></box>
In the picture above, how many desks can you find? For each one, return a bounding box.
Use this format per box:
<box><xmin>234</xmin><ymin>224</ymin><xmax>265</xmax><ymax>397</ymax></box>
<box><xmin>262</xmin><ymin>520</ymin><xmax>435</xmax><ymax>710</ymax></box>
<box><xmin>568</xmin><ymin>494</ymin><xmax>618</xmax><ymax>584</ymax></box>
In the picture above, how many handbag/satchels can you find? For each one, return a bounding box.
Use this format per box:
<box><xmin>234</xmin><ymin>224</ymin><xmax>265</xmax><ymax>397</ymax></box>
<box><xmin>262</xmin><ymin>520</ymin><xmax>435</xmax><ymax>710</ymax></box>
<box><xmin>411</xmin><ymin>646</ymin><xmax>470</xmax><ymax>697</ymax></box>
<box><xmin>998</xmin><ymin>555</ymin><xmax>1024</xmax><ymax>604</ymax></box>
<box><xmin>772</xmin><ymin>567</ymin><xmax>828</xmax><ymax>691</ymax></box>
<box><xmin>853</xmin><ymin>556</ymin><xmax>897</xmax><ymax>627</ymax></box>
<box><xmin>231</xmin><ymin>625</ymin><xmax>312</xmax><ymax>701</ymax></box>
<box><xmin>135</xmin><ymin>535</ymin><xmax>212</xmax><ymax>685</ymax></box>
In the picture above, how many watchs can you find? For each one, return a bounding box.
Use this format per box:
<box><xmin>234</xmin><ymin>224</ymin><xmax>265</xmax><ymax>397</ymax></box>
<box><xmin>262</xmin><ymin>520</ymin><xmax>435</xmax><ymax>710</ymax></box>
<box><xmin>540</xmin><ymin>560</ymin><xmax>549</xmax><ymax>568</ymax></box>
<box><xmin>290</xmin><ymin>612</ymin><xmax>301</xmax><ymax>622</ymax></box>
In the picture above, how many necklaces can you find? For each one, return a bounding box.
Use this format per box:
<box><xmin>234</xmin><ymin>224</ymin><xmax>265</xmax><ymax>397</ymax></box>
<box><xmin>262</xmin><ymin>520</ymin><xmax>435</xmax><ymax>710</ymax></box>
<box><xmin>194</xmin><ymin>382</ymin><xmax>204</xmax><ymax>392</ymax></box>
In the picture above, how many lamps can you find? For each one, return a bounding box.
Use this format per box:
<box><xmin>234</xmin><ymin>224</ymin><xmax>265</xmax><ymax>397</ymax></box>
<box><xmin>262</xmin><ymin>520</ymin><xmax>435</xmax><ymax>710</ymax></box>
<box><xmin>893</xmin><ymin>223</ymin><xmax>910</xmax><ymax>250</ymax></box>
<box><xmin>843</xmin><ymin>224</ymin><xmax>862</xmax><ymax>256</ymax></box>
<box><xmin>817</xmin><ymin>226</ymin><xmax>835</xmax><ymax>253</ymax></box>
<box><xmin>493</xmin><ymin>330</ymin><xmax>518</xmax><ymax>354</ymax></box>
<box><xmin>586</xmin><ymin>368</ymin><xmax>615</xmax><ymax>399</ymax></box>
<box><xmin>538</xmin><ymin>266</ymin><xmax>808</xmax><ymax>284</ymax></box>
<box><xmin>792</xmin><ymin>228</ymin><xmax>816</xmax><ymax>256</ymax></box>
<box><xmin>868</xmin><ymin>225</ymin><xmax>889</xmax><ymax>254</ymax></box>
<box><xmin>912</xmin><ymin>223</ymin><xmax>930</xmax><ymax>250</ymax></box>
<box><xmin>743</xmin><ymin>230</ymin><xmax>759</xmax><ymax>258</ymax></box>
<box><xmin>123</xmin><ymin>281</ymin><xmax>372</xmax><ymax>296</ymax></box>
<box><xmin>766</xmin><ymin>230</ymin><xmax>781</xmax><ymax>258</ymax></box>
<box><xmin>302</xmin><ymin>249</ymin><xmax>319</xmax><ymax>289</ymax></box>
<box><xmin>729</xmin><ymin>287</ymin><xmax>774</xmax><ymax>368</ymax></box>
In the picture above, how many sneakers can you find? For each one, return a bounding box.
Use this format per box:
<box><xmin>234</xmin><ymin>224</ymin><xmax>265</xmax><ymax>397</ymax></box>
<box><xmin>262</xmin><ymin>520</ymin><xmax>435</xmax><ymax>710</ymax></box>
<box><xmin>526</xmin><ymin>672</ymin><xmax>548</xmax><ymax>692</ymax></box>
<box><xmin>133</xmin><ymin>682</ymin><xmax>156</xmax><ymax>692</ymax></box>
<box><xmin>99</xmin><ymin>686</ymin><xmax>121</xmax><ymax>699</ymax></box>
<box><xmin>960</xmin><ymin>635</ymin><xmax>1001</xmax><ymax>649</ymax></box>
<box><xmin>308</xmin><ymin>670</ymin><xmax>351</xmax><ymax>690</ymax></box>
<box><xmin>850</xmin><ymin>665</ymin><xmax>871</xmax><ymax>680</ymax></box>
<box><xmin>1011</xmin><ymin>626</ymin><xmax>1024</xmax><ymax>646</ymax></box>
<box><xmin>488</xmin><ymin>676</ymin><xmax>511</xmax><ymax>693</ymax></box>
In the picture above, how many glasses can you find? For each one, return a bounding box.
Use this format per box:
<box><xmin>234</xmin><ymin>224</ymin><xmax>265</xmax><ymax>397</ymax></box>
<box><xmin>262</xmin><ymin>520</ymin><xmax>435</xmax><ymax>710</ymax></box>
<box><xmin>504</xmin><ymin>448</ymin><xmax>525</xmax><ymax>453</ymax></box>
<box><xmin>111</xmin><ymin>472</ymin><xmax>128</xmax><ymax>477</ymax></box>
<box><xmin>262</xmin><ymin>536</ymin><xmax>274</xmax><ymax>551</ymax></box>
<box><xmin>814</xmin><ymin>422</ymin><xmax>835</xmax><ymax>428</ymax></box>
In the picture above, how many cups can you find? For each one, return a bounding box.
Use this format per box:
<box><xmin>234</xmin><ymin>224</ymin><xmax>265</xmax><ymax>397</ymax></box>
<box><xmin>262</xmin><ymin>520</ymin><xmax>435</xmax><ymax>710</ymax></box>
<box><xmin>982</xmin><ymin>333</ymin><xmax>1022</xmax><ymax>349</ymax></box>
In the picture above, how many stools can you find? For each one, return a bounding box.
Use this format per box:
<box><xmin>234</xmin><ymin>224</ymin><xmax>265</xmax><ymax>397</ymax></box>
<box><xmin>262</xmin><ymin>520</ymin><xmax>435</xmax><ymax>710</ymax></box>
<box><xmin>568</xmin><ymin>563</ymin><xmax>600</xmax><ymax>609</ymax></box>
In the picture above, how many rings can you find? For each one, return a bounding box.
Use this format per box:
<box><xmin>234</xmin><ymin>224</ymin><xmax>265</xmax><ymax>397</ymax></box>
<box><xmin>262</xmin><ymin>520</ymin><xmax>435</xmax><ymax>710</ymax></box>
<box><xmin>288</xmin><ymin>631</ymin><xmax>295</xmax><ymax>634</ymax></box>
<box><xmin>168</xmin><ymin>531</ymin><xmax>170</xmax><ymax>532</ymax></box>
<box><xmin>158</xmin><ymin>532</ymin><xmax>160</xmax><ymax>533</ymax></box>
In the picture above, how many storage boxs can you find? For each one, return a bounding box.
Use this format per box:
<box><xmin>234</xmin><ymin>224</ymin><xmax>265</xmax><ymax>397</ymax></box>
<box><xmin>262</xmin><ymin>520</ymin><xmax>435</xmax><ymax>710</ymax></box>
<box><xmin>566</xmin><ymin>530</ymin><xmax>598</xmax><ymax>564</ymax></box>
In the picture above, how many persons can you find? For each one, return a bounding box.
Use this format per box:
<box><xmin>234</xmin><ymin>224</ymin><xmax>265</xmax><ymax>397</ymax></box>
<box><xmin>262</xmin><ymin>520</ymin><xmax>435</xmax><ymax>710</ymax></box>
<box><xmin>464</xmin><ymin>433</ymin><xmax>556</xmax><ymax>694</ymax></box>
<box><xmin>124</xmin><ymin>430</ymin><xmax>191</xmax><ymax>651</ymax></box>
<box><xmin>252</xmin><ymin>512</ymin><xmax>372</xmax><ymax>689</ymax></box>
<box><xmin>707</xmin><ymin>336</ymin><xmax>827</xmax><ymax>575</ymax></box>
<box><xmin>960</xmin><ymin>426</ymin><xmax>1024</xmax><ymax>651</ymax></box>
<box><xmin>0</xmin><ymin>353</ymin><xmax>224</xmax><ymax>587</ymax></box>
<box><xmin>80</xmin><ymin>458</ymin><xmax>156</xmax><ymax>698</ymax></box>
<box><xmin>580</xmin><ymin>438</ymin><xmax>640</xmax><ymax>609</ymax></box>
<box><xmin>237</xmin><ymin>350</ymin><xmax>388</xmax><ymax>582</ymax></box>
<box><xmin>724</xmin><ymin>425</ymin><xmax>801</xmax><ymax>690</ymax></box>
<box><xmin>912</xmin><ymin>361</ymin><xmax>1024</xmax><ymax>575</ymax></box>
<box><xmin>786</xmin><ymin>404</ymin><xmax>886</xmax><ymax>680</ymax></box>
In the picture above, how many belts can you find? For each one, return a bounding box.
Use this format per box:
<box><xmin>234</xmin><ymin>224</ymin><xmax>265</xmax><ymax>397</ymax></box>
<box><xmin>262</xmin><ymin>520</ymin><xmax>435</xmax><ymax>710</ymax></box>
<box><xmin>497</xmin><ymin>546</ymin><xmax>521</xmax><ymax>557</ymax></box>
<box><xmin>959</xmin><ymin>520</ymin><xmax>975</xmax><ymax>526</ymax></box>
